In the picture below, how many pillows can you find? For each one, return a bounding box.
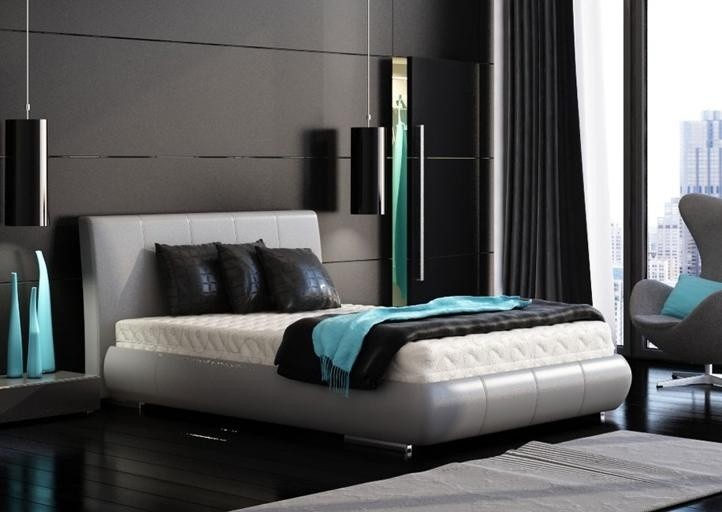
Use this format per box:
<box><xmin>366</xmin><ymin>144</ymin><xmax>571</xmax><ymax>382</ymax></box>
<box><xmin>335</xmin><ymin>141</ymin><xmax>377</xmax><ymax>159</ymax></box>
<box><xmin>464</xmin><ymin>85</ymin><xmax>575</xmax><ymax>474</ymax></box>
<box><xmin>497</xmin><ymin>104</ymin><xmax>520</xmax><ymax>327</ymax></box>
<box><xmin>152</xmin><ymin>237</ymin><xmax>342</xmax><ymax>318</ymax></box>
<box><xmin>660</xmin><ymin>274</ymin><xmax>722</xmax><ymax>321</ymax></box>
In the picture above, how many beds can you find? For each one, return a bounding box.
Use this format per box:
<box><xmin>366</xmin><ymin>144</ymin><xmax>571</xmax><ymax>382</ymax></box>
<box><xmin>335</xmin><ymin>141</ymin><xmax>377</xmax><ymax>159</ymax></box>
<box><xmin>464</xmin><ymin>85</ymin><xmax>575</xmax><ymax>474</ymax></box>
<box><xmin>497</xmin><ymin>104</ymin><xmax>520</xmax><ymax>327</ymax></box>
<box><xmin>77</xmin><ymin>208</ymin><xmax>634</xmax><ymax>459</ymax></box>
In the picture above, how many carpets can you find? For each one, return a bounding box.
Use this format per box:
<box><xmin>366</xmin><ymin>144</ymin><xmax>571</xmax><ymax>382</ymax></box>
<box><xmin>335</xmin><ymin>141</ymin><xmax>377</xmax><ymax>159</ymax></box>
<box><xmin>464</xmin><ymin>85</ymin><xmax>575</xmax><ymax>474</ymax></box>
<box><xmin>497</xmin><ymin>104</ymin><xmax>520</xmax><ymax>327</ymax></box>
<box><xmin>227</xmin><ymin>429</ymin><xmax>722</xmax><ymax>511</ymax></box>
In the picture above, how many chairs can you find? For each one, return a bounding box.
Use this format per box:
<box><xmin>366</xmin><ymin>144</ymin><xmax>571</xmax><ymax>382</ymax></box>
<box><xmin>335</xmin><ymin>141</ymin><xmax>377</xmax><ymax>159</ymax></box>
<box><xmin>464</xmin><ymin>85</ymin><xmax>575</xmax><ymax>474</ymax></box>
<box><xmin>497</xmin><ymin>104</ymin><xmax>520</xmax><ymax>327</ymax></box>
<box><xmin>625</xmin><ymin>194</ymin><xmax>722</xmax><ymax>391</ymax></box>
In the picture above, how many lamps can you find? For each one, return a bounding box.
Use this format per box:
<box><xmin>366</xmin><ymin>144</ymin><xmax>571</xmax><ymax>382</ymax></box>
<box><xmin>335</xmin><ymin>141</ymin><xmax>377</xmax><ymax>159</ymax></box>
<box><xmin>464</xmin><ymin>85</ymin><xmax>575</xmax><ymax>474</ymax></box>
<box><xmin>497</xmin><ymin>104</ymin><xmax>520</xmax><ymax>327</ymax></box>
<box><xmin>5</xmin><ymin>0</ymin><xmax>51</xmax><ymax>228</ymax></box>
<box><xmin>348</xmin><ymin>2</ymin><xmax>389</xmax><ymax>214</ymax></box>
